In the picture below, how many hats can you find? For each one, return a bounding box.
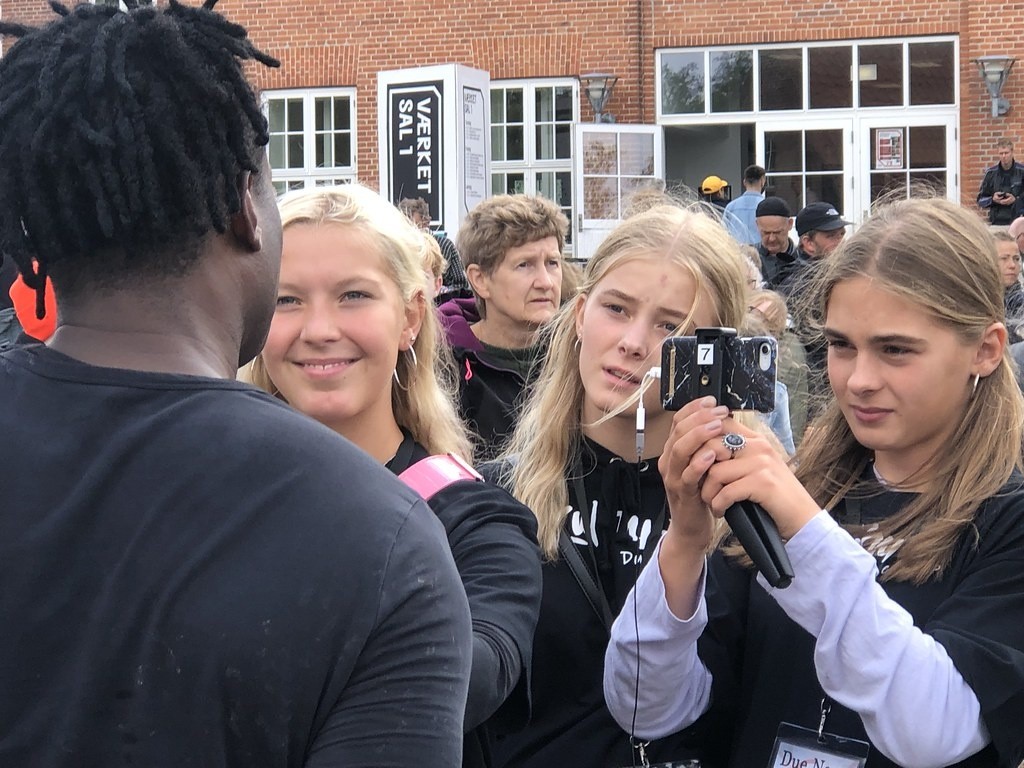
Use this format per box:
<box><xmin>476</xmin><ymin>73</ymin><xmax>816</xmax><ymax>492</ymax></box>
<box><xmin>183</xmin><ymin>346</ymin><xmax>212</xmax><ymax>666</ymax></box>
<box><xmin>702</xmin><ymin>175</ymin><xmax>728</xmax><ymax>194</ymax></box>
<box><xmin>757</xmin><ymin>195</ymin><xmax>791</xmax><ymax>216</ymax></box>
<box><xmin>794</xmin><ymin>201</ymin><xmax>855</xmax><ymax>237</ymax></box>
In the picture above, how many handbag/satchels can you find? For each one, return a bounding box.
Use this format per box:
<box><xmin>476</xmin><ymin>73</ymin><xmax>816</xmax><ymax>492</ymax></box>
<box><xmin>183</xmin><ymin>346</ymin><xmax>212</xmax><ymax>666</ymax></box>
<box><xmin>465</xmin><ymin>532</ymin><xmax>697</xmax><ymax>766</ymax></box>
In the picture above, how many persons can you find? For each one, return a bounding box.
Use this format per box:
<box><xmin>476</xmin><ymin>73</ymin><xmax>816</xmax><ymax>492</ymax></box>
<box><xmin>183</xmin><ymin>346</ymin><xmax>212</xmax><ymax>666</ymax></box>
<box><xmin>463</xmin><ymin>204</ymin><xmax>777</xmax><ymax>768</ymax></box>
<box><xmin>604</xmin><ymin>197</ymin><xmax>1023</xmax><ymax>768</ymax></box>
<box><xmin>236</xmin><ymin>181</ymin><xmax>543</xmax><ymax>768</ymax></box>
<box><xmin>1</xmin><ymin>0</ymin><xmax>473</xmax><ymax>767</ymax></box>
<box><xmin>396</xmin><ymin>136</ymin><xmax>1024</xmax><ymax>460</ymax></box>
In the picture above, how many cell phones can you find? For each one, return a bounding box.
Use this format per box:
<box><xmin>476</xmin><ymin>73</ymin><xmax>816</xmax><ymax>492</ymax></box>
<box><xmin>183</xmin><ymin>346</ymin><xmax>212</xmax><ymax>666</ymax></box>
<box><xmin>662</xmin><ymin>335</ymin><xmax>777</xmax><ymax>414</ymax></box>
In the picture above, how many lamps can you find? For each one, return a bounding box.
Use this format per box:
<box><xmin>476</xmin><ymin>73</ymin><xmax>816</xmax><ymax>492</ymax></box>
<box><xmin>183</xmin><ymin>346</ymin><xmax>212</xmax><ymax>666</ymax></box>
<box><xmin>574</xmin><ymin>73</ymin><xmax>621</xmax><ymax>123</ymax></box>
<box><xmin>970</xmin><ymin>53</ymin><xmax>1021</xmax><ymax>120</ymax></box>
